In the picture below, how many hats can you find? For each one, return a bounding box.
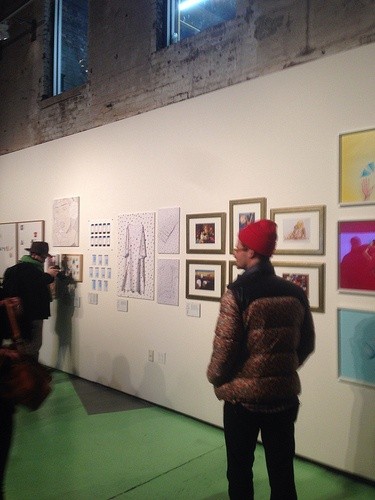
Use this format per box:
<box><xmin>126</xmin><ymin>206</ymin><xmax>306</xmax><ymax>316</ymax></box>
<box><xmin>25</xmin><ymin>242</ymin><xmax>52</xmax><ymax>258</ymax></box>
<box><xmin>237</xmin><ymin>219</ymin><xmax>277</xmax><ymax>256</ymax></box>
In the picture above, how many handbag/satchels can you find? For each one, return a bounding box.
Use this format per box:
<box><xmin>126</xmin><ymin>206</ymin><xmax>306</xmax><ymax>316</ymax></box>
<box><xmin>12</xmin><ymin>358</ymin><xmax>53</xmax><ymax>411</ymax></box>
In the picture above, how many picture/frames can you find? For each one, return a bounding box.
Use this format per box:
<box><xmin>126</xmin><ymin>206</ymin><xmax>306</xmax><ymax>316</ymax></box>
<box><xmin>185</xmin><ymin>260</ymin><xmax>226</xmax><ymax>302</ymax></box>
<box><xmin>229</xmin><ymin>197</ymin><xmax>268</xmax><ymax>255</ymax></box>
<box><xmin>338</xmin><ymin>126</ymin><xmax>375</xmax><ymax>207</ymax></box>
<box><xmin>229</xmin><ymin>260</ymin><xmax>246</xmax><ymax>282</ymax></box>
<box><xmin>336</xmin><ymin>217</ymin><xmax>375</xmax><ymax>296</ymax></box>
<box><xmin>272</xmin><ymin>261</ymin><xmax>325</xmax><ymax>314</ymax></box>
<box><xmin>61</xmin><ymin>254</ymin><xmax>82</xmax><ymax>282</ymax></box>
<box><xmin>335</xmin><ymin>304</ymin><xmax>375</xmax><ymax>390</ymax></box>
<box><xmin>270</xmin><ymin>205</ymin><xmax>326</xmax><ymax>256</ymax></box>
<box><xmin>186</xmin><ymin>213</ymin><xmax>225</xmax><ymax>255</ymax></box>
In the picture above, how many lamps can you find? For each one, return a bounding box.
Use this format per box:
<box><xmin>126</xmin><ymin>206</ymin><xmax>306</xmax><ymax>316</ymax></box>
<box><xmin>0</xmin><ymin>21</ymin><xmax>9</xmax><ymax>41</ymax></box>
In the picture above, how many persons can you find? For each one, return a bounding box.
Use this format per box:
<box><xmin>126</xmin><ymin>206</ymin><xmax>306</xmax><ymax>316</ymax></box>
<box><xmin>200</xmin><ymin>226</ymin><xmax>212</xmax><ymax>242</ymax></box>
<box><xmin>0</xmin><ymin>288</ymin><xmax>26</xmax><ymax>500</ymax></box>
<box><xmin>207</xmin><ymin>220</ymin><xmax>315</xmax><ymax>500</ymax></box>
<box><xmin>17</xmin><ymin>242</ymin><xmax>59</xmax><ymax>362</ymax></box>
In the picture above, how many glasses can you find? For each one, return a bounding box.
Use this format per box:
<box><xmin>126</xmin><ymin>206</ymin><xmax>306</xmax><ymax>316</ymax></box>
<box><xmin>231</xmin><ymin>248</ymin><xmax>246</xmax><ymax>256</ymax></box>
